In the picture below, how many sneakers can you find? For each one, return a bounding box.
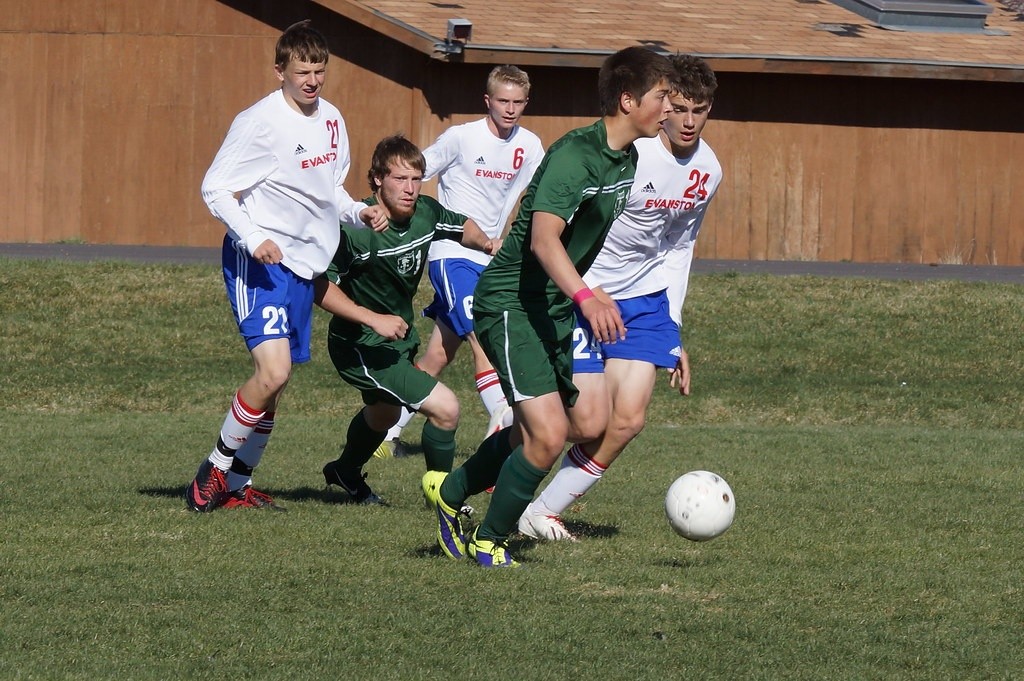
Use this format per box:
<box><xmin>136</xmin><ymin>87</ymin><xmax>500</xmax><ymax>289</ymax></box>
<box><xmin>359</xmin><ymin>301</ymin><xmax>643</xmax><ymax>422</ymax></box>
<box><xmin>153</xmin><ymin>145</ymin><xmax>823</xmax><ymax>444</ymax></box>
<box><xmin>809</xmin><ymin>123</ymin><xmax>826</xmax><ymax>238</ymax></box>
<box><xmin>518</xmin><ymin>503</ymin><xmax>581</xmax><ymax>543</ymax></box>
<box><xmin>186</xmin><ymin>457</ymin><xmax>228</xmax><ymax>513</ymax></box>
<box><xmin>420</xmin><ymin>470</ymin><xmax>468</xmax><ymax>559</ymax></box>
<box><xmin>219</xmin><ymin>486</ymin><xmax>287</xmax><ymax>513</ymax></box>
<box><xmin>467</xmin><ymin>526</ymin><xmax>522</xmax><ymax>568</ymax></box>
<box><xmin>322</xmin><ymin>459</ymin><xmax>386</xmax><ymax>506</ymax></box>
<box><xmin>372</xmin><ymin>437</ymin><xmax>410</xmax><ymax>459</ymax></box>
<box><xmin>423</xmin><ymin>496</ymin><xmax>478</xmax><ymax>516</ymax></box>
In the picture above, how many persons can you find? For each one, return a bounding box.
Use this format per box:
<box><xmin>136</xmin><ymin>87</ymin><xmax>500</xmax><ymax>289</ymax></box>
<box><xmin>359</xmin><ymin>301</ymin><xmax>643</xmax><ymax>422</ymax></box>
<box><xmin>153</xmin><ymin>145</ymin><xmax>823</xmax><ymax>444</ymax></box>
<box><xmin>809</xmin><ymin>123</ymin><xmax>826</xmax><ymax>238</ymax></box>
<box><xmin>484</xmin><ymin>54</ymin><xmax>724</xmax><ymax>543</ymax></box>
<box><xmin>372</xmin><ymin>65</ymin><xmax>547</xmax><ymax>492</ymax></box>
<box><xmin>315</xmin><ymin>134</ymin><xmax>505</xmax><ymax>521</ymax></box>
<box><xmin>184</xmin><ymin>20</ymin><xmax>393</xmax><ymax>517</ymax></box>
<box><xmin>418</xmin><ymin>43</ymin><xmax>678</xmax><ymax>566</ymax></box>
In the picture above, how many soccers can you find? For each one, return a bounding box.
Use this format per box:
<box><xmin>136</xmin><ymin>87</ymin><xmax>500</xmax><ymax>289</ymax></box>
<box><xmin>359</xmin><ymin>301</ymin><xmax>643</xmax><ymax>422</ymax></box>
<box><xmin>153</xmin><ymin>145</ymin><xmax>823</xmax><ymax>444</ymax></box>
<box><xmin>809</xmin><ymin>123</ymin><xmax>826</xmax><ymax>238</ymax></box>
<box><xmin>666</xmin><ymin>470</ymin><xmax>734</xmax><ymax>542</ymax></box>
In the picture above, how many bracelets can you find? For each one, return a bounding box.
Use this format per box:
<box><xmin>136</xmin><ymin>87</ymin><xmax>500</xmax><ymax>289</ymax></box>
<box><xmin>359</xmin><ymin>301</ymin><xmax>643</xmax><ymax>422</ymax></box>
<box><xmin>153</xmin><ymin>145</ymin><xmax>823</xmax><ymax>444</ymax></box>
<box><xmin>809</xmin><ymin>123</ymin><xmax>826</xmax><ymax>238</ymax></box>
<box><xmin>571</xmin><ymin>287</ymin><xmax>593</xmax><ymax>305</ymax></box>
